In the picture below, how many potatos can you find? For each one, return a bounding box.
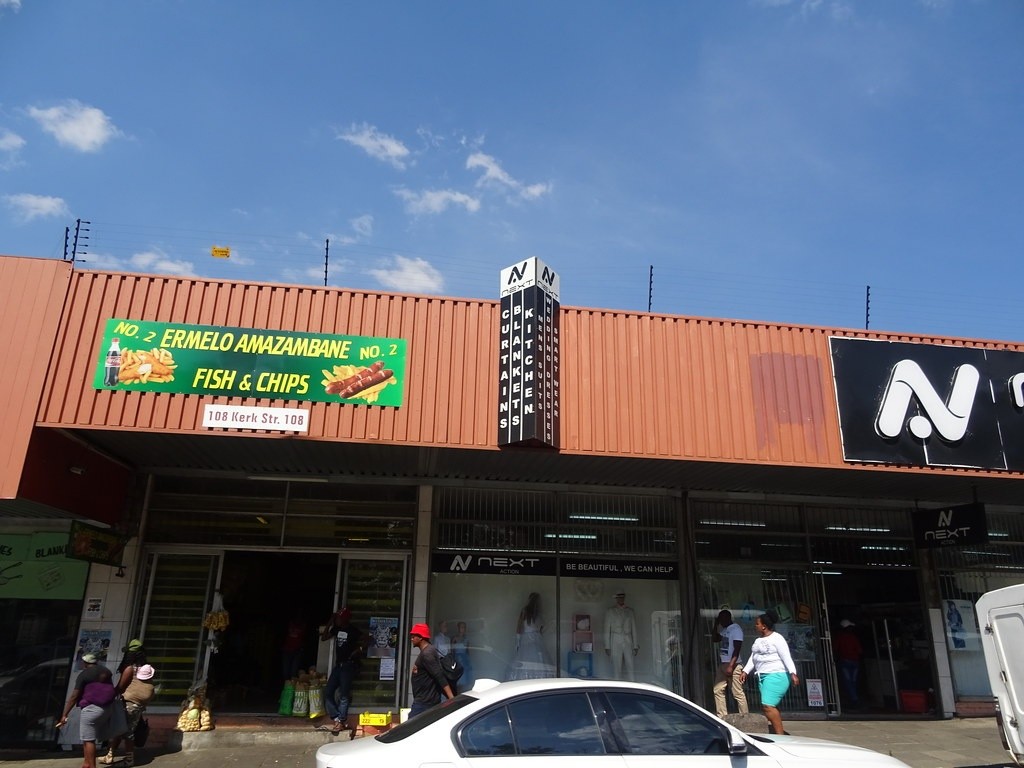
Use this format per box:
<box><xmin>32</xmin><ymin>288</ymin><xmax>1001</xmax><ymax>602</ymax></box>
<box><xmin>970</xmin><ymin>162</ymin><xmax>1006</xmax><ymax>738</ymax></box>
<box><xmin>177</xmin><ymin>698</ymin><xmax>213</xmax><ymax>732</ymax></box>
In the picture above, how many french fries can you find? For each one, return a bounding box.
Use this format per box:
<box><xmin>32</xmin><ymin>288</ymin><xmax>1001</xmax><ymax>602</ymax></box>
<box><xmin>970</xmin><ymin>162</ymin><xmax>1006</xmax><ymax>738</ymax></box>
<box><xmin>119</xmin><ymin>347</ymin><xmax>178</xmax><ymax>383</ymax></box>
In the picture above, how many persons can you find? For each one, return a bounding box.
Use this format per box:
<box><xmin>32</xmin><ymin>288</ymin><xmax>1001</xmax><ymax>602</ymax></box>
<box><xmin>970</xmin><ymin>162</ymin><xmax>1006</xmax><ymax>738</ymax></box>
<box><xmin>321</xmin><ymin>608</ymin><xmax>362</xmax><ymax>731</ymax></box>
<box><xmin>506</xmin><ymin>593</ymin><xmax>556</xmax><ymax>680</ymax></box>
<box><xmin>452</xmin><ymin>622</ymin><xmax>474</xmax><ymax>692</ymax></box>
<box><xmin>739</xmin><ymin>611</ymin><xmax>799</xmax><ymax>735</ymax></box>
<box><xmin>272</xmin><ymin>607</ymin><xmax>314</xmax><ymax>704</ymax></box>
<box><xmin>837</xmin><ymin>620</ymin><xmax>863</xmax><ymax>708</ymax></box>
<box><xmin>712</xmin><ymin>610</ymin><xmax>748</xmax><ymax>715</ymax></box>
<box><xmin>604</xmin><ymin>590</ymin><xmax>640</xmax><ymax>682</ymax></box>
<box><xmin>61</xmin><ymin>640</ymin><xmax>155</xmax><ymax>768</ymax></box>
<box><xmin>434</xmin><ymin>621</ymin><xmax>451</xmax><ymax>660</ymax></box>
<box><xmin>409</xmin><ymin>623</ymin><xmax>454</xmax><ymax>718</ymax></box>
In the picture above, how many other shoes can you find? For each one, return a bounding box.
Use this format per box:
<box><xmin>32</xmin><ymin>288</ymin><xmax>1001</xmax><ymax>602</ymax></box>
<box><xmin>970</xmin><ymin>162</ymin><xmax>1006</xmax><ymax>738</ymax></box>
<box><xmin>96</xmin><ymin>755</ymin><xmax>113</xmax><ymax>765</ymax></box>
<box><xmin>332</xmin><ymin>719</ymin><xmax>346</xmax><ymax>732</ymax></box>
<box><xmin>114</xmin><ymin>758</ymin><xmax>135</xmax><ymax>767</ymax></box>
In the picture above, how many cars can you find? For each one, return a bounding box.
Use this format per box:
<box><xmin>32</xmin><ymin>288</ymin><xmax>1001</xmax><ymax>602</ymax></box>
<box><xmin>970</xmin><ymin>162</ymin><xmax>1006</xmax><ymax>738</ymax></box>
<box><xmin>0</xmin><ymin>657</ymin><xmax>73</xmax><ymax>751</ymax></box>
<box><xmin>315</xmin><ymin>677</ymin><xmax>915</xmax><ymax>768</ymax></box>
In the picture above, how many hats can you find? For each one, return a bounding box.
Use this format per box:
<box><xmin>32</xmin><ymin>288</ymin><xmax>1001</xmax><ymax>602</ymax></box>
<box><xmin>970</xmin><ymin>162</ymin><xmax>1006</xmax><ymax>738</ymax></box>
<box><xmin>410</xmin><ymin>623</ymin><xmax>431</xmax><ymax>638</ymax></box>
<box><xmin>613</xmin><ymin>591</ymin><xmax>625</xmax><ymax>598</ymax></box>
<box><xmin>840</xmin><ymin>620</ymin><xmax>855</xmax><ymax>627</ymax></box>
<box><xmin>82</xmin><ymin>654</ymin><xmax>97</xmax><ymax>663</ymax></box>
<box><xmin>137</xmin><ymin>664</ymin><xmax>155</xmax><ymax>680</ymax></box>
<box><xmin>334</xmin><ymin>607</ymin><xmax>351</xmax><ymax>620</ymax></box>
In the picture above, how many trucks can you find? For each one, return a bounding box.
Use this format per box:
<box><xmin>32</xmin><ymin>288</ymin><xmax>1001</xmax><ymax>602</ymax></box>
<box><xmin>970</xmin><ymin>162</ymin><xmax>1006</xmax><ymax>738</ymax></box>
<box><xmin>974</xmin><ymin>583</ymin><xmax>1023</xmax><ymax>768</ymax></box>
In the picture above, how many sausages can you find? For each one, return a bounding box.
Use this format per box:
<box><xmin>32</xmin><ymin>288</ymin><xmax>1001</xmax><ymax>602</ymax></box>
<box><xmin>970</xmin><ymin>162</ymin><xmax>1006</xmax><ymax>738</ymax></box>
<box><xmin>325</xmin><ymin>361</ymin><xmax>393</xmax><ymax>398</ymax></box>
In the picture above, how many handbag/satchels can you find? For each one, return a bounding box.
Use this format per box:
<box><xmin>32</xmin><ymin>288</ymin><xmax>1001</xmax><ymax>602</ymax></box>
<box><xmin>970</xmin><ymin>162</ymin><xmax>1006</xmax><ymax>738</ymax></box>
<box><xmin>104</xmin><ymin>693</ymin><xmax>128</xmax><ymax>737</ymax></box>
<box><xmin>436</xmin><ymin>649</ymin><xmax>463</xmax><ymax>698</ymax></box>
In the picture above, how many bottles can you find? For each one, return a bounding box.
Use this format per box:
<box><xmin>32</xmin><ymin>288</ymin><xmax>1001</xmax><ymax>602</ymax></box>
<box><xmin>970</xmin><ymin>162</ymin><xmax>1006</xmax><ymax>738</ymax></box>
<box><xmin>104</xmin><ymin>339</ymin><xmax>121</xmax><ymax>386</ymax></box>
